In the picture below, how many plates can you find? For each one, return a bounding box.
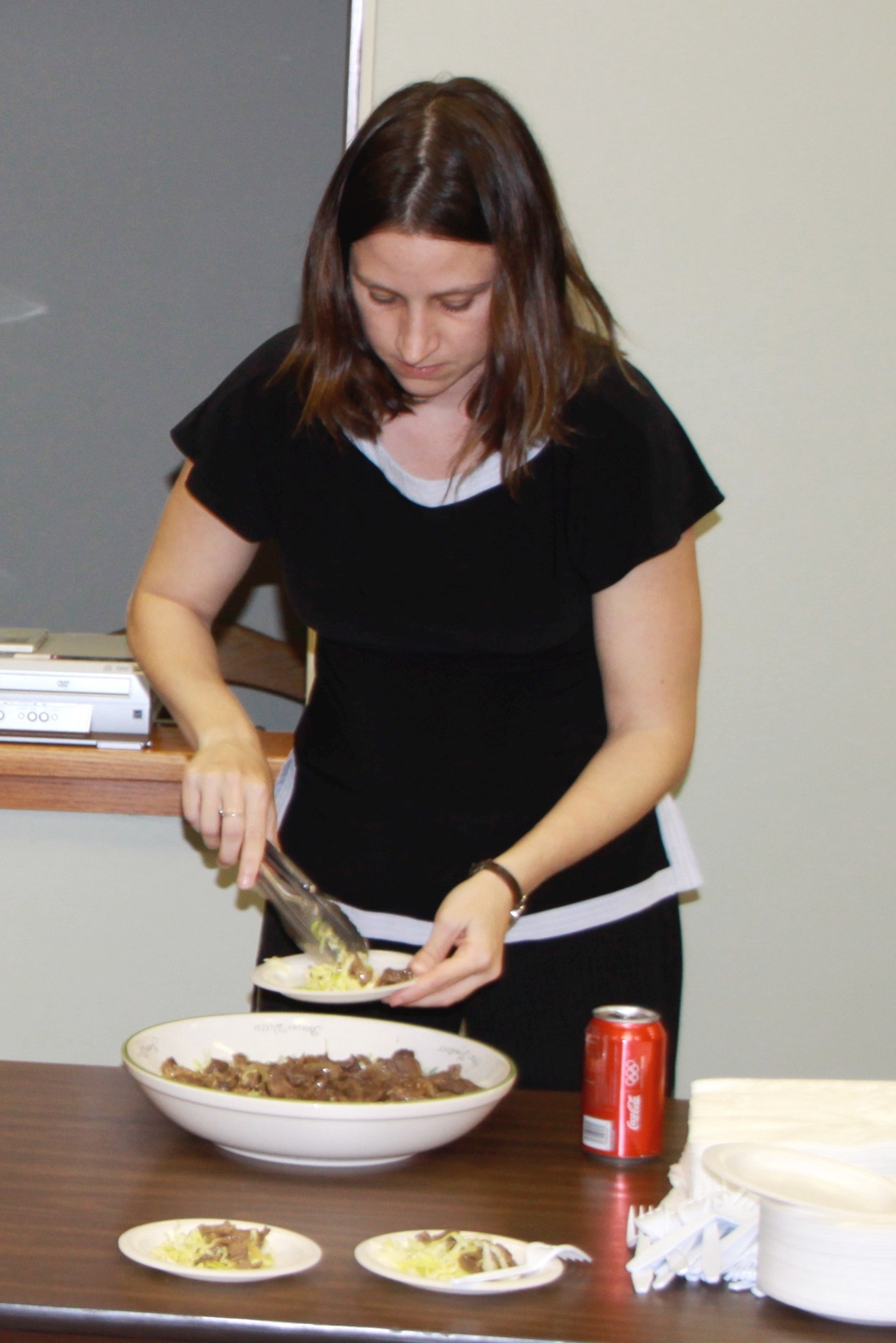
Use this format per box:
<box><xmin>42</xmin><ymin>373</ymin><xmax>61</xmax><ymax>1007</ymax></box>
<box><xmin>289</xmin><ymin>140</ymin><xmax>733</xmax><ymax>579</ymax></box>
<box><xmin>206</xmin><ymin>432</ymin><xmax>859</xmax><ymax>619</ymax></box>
<box><xmin>117</xmin><ymin>1219</ymin><xmax>321</xmax><ymax>1282</ymax></box>
<box><xmin>353</xmin><ymin>1228</ymin><xmax>563</xmax><ymax>1295</ymax></box>
<box><xmin>249</xmin><ymin>949</ymin><xmax>417</xmax><ymax>1003</ymax></box>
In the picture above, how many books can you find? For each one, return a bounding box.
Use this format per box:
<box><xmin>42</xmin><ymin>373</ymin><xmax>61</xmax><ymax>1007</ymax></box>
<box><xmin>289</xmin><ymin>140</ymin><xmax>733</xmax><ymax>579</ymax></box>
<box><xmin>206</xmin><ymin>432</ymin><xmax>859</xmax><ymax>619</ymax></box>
<box><xmin>0</xmin><ymin>627</ymin><xmax>138</xmax><ymax>660</ymax></box>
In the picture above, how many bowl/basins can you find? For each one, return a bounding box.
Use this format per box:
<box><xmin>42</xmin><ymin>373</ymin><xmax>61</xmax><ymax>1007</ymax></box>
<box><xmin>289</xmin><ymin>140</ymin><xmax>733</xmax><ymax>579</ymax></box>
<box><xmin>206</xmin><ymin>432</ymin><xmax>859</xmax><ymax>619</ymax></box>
<box><xmin>122</xmin><ymin>1010</ymin><xmax>517</xmax><ymax>1170</ymax></box>
<box><xmin>701</xmin><ymin>1139</ymin><xmax>896</xmax><ymax>1328</ymax></box>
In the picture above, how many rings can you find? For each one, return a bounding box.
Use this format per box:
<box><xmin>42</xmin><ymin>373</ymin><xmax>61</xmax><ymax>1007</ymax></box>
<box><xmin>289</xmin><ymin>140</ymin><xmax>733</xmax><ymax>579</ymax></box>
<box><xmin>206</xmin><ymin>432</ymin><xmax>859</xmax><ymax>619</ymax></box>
<box><xmin>219</xmin><ymin>810</ymin><xmax>245</xmax><ymax>817</ymax></box>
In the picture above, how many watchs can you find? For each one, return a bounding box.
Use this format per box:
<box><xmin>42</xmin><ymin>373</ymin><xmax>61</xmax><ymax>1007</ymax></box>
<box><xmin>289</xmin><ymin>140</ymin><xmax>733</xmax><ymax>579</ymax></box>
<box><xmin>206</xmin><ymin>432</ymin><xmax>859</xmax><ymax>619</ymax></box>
<box><xmin>468</xmin><ymin>857</ymin><xmax>527</xmax><ymax>929</ymax></box>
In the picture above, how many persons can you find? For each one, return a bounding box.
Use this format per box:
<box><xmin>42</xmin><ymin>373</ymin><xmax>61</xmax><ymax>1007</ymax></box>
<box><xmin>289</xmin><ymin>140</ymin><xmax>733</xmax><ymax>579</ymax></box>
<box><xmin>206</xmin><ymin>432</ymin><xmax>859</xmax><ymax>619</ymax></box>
<box><xmin>126</xmin><ymin>77</ymin><xmax>728</xmax><ymax>1097</ymax></box>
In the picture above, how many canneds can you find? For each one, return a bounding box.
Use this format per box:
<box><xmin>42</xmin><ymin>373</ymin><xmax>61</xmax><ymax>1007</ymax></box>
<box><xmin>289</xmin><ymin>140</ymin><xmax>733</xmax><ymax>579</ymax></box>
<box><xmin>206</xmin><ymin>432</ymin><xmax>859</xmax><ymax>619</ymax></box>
<box><xmin>582</xmin><ymin>1004</ymin><xmax>669</xmax><ymax>1164</ymax></box>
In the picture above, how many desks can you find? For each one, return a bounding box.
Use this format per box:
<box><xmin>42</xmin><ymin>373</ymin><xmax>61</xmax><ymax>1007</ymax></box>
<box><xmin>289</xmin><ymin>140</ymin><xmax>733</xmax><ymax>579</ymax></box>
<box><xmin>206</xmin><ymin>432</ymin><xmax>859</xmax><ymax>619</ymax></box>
<box><xmin>1</xmin><ymin>1061</ymin><xmax>895</xmax><ymax>1343</ymax></box>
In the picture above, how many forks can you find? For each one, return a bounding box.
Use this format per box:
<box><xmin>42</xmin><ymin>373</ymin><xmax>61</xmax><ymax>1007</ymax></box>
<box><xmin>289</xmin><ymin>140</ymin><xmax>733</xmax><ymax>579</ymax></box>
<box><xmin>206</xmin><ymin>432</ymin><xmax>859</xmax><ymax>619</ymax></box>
<box><xmin>623</xmin><ymin>1204</ymin><xmax>654</xmax><ymax>1292</ymax></box>
<box><xmin>450</xmin><ymin>1241</ymin><xmax>592</xmax><ymax>1285</ymax></box>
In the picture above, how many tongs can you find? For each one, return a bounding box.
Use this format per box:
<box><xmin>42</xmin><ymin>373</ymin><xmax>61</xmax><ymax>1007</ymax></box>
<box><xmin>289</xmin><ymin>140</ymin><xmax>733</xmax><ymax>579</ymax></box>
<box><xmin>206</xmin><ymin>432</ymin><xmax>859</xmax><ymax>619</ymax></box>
<box><xmin>255</xmin><ymin>839</ymin><xmax>373</xmax><ymax>985</ymax></box>
